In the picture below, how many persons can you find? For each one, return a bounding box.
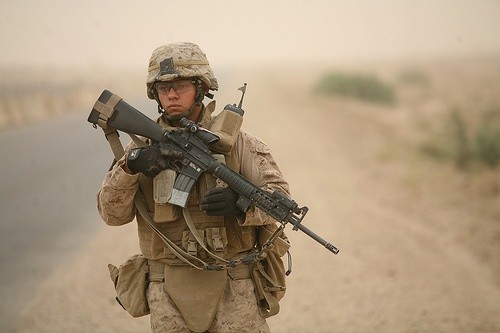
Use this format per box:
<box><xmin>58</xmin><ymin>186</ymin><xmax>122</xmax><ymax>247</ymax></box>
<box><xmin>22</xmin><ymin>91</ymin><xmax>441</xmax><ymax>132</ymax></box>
<box><xmin>98</xmin><ymin>41</ymin><xmax>291</xmax><ymax>333</ymax></box>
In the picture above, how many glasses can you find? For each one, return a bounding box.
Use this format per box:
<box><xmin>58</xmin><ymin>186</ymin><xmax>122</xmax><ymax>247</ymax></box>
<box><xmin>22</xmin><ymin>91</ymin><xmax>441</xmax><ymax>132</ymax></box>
<box><xmin>151</xmin><ymin>79</ymin><xmax>197</xmax><ymax>96</ymax></box>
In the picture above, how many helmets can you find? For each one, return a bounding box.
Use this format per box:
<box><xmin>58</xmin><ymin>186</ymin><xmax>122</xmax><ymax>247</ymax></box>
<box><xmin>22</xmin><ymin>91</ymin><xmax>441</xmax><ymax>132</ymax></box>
<box><xmin>144</xmin><ymin>42</ymin><xmax>218</xmax><ymax>98</ymax></box>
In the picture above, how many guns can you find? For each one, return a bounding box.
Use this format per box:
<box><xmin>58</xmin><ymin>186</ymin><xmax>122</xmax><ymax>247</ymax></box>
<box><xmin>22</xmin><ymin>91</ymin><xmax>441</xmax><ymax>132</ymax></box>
<box><xmin>86</xmin><ymin>89</ymin><xmax>339</xmax><ymax>255</ymax></box>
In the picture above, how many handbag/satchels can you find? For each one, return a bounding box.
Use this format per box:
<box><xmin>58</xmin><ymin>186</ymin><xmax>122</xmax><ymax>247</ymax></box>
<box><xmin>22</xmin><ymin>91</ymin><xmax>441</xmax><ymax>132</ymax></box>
<box><xmin>248</xmin><ymin>221</ymin><xmax>291</xmax><ymax>305</ymax></box>
<box><xmin>106</xmin><ymin>253</ymin><xmax>152</xmax><ymax>317</ymax></box>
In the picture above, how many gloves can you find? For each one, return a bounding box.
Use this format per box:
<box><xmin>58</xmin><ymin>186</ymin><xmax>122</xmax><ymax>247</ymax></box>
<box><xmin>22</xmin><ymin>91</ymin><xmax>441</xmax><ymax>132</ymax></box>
<box><xmin>127</xmin><ymin>146</ymin><xmax>161</xmax><ymax>176</ymax></box>
<box><xmin>200</xmin><ymin>186</ymin><xmax>243</xmax><ymax>217</ymax></box>
